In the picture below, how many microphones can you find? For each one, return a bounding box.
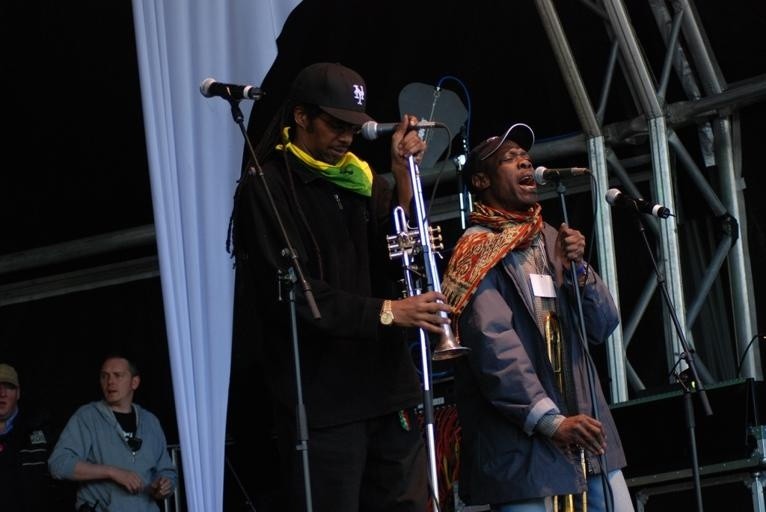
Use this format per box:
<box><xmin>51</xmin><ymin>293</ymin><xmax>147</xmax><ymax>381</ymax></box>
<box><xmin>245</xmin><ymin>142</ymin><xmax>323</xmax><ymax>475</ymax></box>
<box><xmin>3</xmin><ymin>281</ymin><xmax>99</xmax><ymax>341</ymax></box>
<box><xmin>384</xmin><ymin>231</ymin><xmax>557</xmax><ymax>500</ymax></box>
<box><xmin>362</xmin><ymin>119</ymin><xmax>436</xmax><ymax>143</ymax></box>
<box><xmin>606</xmin><ymin>187</ymin><xmax>672</xmax><ymax>218</ymax></box>
<box><xmin>535</xmin><ymin>164</ymin><xmax>596</xmax><ymax>187</ymax></box>
<box><xmin>201</xmin><ymin>77</ymin><xmax>268</xmax><ymax>101</ymax></box>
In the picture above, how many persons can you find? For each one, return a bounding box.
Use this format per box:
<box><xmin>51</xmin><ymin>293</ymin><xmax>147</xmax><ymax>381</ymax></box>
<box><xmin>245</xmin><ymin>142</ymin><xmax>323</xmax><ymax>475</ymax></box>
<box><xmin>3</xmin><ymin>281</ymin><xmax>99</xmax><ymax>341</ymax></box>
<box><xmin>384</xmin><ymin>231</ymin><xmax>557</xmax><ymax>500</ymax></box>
<box><xmin>220</xmin><ymin>60</ymin><xmax>455</xmax><ymax>512</ymax></box>
<box><xmin>440</xmin><ymin>123</ymin><xmax>639</xmax><ymax>512</ymax></box>
<box><xmin>46</xmin><ymin>353</ymin><xmax>180</xmax><ymax>511</ymax></box>
<box><xmin>1</xmin><ymin>363</ymin><xmax>52</xmax><ymax>511</ymax></box>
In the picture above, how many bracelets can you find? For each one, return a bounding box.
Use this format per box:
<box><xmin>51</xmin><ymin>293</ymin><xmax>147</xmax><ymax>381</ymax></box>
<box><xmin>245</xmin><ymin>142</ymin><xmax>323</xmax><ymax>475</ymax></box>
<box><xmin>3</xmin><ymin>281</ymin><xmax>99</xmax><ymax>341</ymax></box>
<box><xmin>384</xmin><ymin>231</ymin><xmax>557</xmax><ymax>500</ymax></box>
<box><xmin>564</xmin><ymin>259</ymin><xmax>592</xmax><ymax>281</ymax></box>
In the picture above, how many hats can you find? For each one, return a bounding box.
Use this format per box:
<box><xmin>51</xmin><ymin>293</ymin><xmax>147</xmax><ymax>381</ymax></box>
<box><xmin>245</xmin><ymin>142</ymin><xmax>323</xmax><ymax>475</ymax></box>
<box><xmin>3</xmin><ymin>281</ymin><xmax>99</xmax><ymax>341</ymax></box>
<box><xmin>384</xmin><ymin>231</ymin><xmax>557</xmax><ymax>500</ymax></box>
<box><xmin>0</xmin><ymin>364</ymin><xmax>20</xmax><ymax>387</ymax></box>
<box><xmin>294</xmin><ymin>62</ymin><xmax>374</xmax><ymax>126</ymax></box>
<box><xmin>462</xmin><ymin>123</ymin><xmax>535</xmax><ymax>194</ymax></box>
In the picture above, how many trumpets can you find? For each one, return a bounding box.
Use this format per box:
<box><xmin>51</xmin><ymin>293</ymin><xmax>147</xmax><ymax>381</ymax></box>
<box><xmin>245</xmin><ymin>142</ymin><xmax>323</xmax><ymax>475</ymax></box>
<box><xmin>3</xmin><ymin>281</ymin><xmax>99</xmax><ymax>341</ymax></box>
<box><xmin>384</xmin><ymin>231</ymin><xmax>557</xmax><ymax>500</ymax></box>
<box><xmin>386</xmin><ymin>155</ymin><xmax>471</xmax><ymax>359</ymax></box>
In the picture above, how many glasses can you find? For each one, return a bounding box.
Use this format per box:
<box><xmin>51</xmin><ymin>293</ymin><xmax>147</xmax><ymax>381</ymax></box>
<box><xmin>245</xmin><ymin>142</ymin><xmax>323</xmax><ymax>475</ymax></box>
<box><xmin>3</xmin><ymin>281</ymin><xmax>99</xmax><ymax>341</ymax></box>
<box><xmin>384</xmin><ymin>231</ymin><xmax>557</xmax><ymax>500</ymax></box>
<box><xmin>319</xmin><ymin>116</ymin><xmax>361</xmax><ymax>134</ymax></box>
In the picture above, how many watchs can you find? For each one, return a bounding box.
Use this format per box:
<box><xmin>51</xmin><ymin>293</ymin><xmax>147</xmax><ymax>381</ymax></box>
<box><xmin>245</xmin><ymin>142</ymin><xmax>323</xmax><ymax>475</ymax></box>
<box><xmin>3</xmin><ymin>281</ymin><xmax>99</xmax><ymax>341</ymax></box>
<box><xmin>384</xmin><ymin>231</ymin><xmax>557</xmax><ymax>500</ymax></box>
<box><xmin>378</xmin><ymin>299</ymin><xmax>395</xmax><ymax>328</ymax></box>
